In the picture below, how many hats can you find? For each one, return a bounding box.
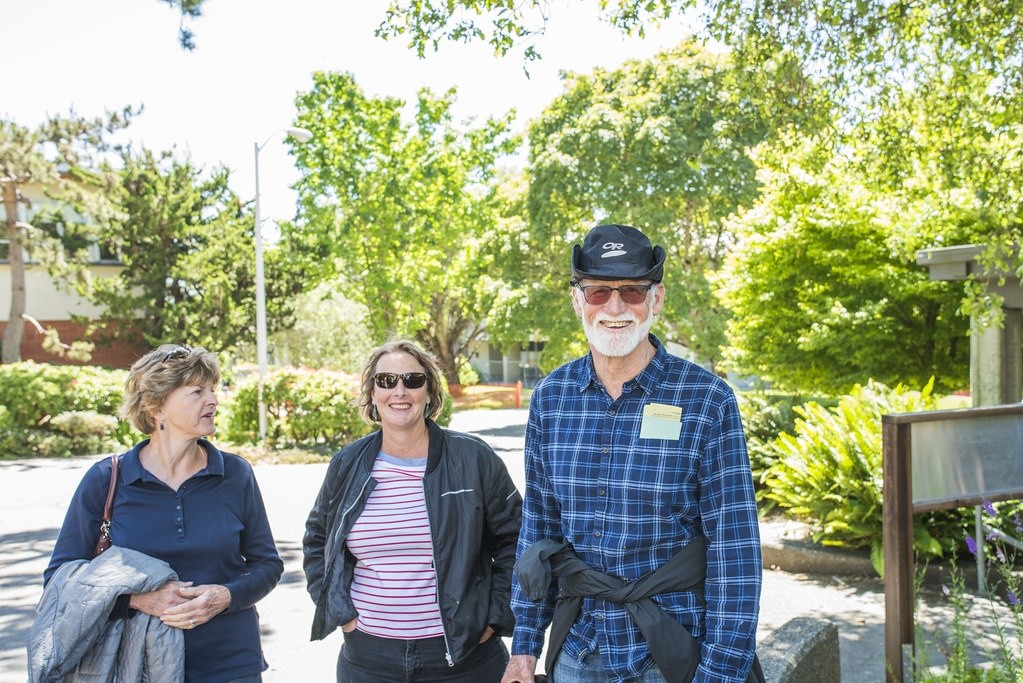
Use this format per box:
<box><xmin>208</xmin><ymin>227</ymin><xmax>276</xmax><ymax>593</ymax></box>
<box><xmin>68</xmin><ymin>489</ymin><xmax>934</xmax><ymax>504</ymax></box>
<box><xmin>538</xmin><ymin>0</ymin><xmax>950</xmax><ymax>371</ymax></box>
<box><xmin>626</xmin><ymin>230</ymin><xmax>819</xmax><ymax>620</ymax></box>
<box><xmin>568</xmin><ymin>225</ymin><xmax>666</xmax><ymax>286</ymax></box>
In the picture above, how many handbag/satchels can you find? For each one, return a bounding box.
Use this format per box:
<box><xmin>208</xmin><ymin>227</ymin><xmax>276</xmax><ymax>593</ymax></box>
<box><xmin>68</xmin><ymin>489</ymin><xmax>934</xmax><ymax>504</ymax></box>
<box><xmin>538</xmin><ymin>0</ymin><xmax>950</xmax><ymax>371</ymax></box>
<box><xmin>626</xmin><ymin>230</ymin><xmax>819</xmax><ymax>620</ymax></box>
<box><xmin>93</xmin><ymin>454</ymin><xmax>118</xmax><ymax>558</ymax></box>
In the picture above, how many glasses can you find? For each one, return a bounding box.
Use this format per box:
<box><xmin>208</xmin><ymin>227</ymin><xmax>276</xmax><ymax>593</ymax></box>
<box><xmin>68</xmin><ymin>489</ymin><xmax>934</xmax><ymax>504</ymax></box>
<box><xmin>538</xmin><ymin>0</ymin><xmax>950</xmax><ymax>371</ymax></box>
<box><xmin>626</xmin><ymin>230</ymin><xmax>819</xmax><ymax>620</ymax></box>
<box><xmin>576</xmin><ymin>280</ymin><xmax>655</xmax><ymax>305</ymax></box>
<box><xmin>372</xmin><ymin>372</ymin><xmax>431</xmax><ymax>388</ymax></box>
<box><xmin>161</xmin><ymin>345</ymin><xmax>194</xmax><ymax>363</ymax></box>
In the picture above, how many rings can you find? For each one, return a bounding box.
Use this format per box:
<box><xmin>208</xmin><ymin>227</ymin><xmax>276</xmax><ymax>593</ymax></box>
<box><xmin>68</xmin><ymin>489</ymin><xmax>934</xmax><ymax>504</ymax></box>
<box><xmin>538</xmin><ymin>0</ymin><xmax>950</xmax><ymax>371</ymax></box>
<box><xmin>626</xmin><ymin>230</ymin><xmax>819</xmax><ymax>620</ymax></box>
<box><xmin>189</xmin><ymin>620</ymin><xmax>194</xmax><ymax>628</ymax></box>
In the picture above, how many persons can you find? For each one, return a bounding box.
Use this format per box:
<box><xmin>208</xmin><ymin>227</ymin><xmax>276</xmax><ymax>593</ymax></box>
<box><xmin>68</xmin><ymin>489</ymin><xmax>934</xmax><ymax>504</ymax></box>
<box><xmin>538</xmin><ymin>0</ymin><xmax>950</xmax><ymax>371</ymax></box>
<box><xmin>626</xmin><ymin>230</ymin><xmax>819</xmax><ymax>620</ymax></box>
<box><xmin>499</xmin><ymin>225</ymin><xmax>767</xmax><ymax>683</ymax></box>
<box><xmin>43</xmin><ymin>344</ymin><xmax>284</xmax><ymax>683</ymax></box>
<box><xmin>303</xmin><ymin>339</ymin><xmax>524</xmax><ymax>683</ymax></box>
